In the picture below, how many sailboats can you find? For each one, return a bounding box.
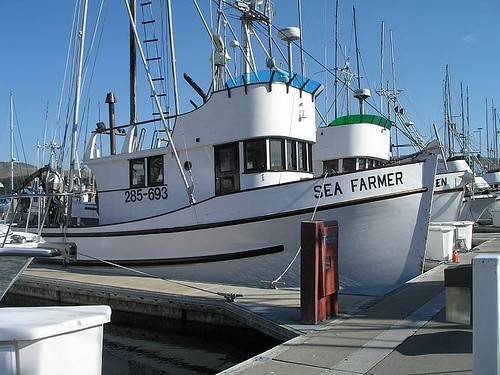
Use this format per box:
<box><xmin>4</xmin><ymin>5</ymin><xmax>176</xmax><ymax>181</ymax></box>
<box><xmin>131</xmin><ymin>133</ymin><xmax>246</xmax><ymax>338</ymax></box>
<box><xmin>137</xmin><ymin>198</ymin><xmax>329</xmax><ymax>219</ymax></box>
<box><xmin>0</xmin><ymin>0</ymin><xmax>438</xmax><ymax>290</ymax></box>
<box><xmin>306</xmin><ymin>0</ymin><xmax>475</xmax><ymax>267</ymax></box>
<box><xmin>374</xmin><ymin>16</ymin><xmax>498</xmax><ymax>227</ymax></box>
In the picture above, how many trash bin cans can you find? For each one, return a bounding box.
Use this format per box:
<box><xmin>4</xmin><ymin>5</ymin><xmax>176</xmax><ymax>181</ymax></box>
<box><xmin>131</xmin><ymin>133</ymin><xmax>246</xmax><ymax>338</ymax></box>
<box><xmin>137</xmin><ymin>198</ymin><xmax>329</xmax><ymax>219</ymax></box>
<box><xmin>425</xmin><ymin>226</ymin><xmax>456</xmax><ymax>261</ymax></box>
<box><xmin>444</xmin><ymin>264</ymin><xmax>473</xmax><ymax>327</ymax></box>
<box><xmin>1</xmin><ymin>304</ymin><xmax>112</xmax><ymax>375</ymax></box>
<box><xmin>429</xmin><ymin>221</ymin><xmax>474</xmax><ymax>252</ymax></box>
